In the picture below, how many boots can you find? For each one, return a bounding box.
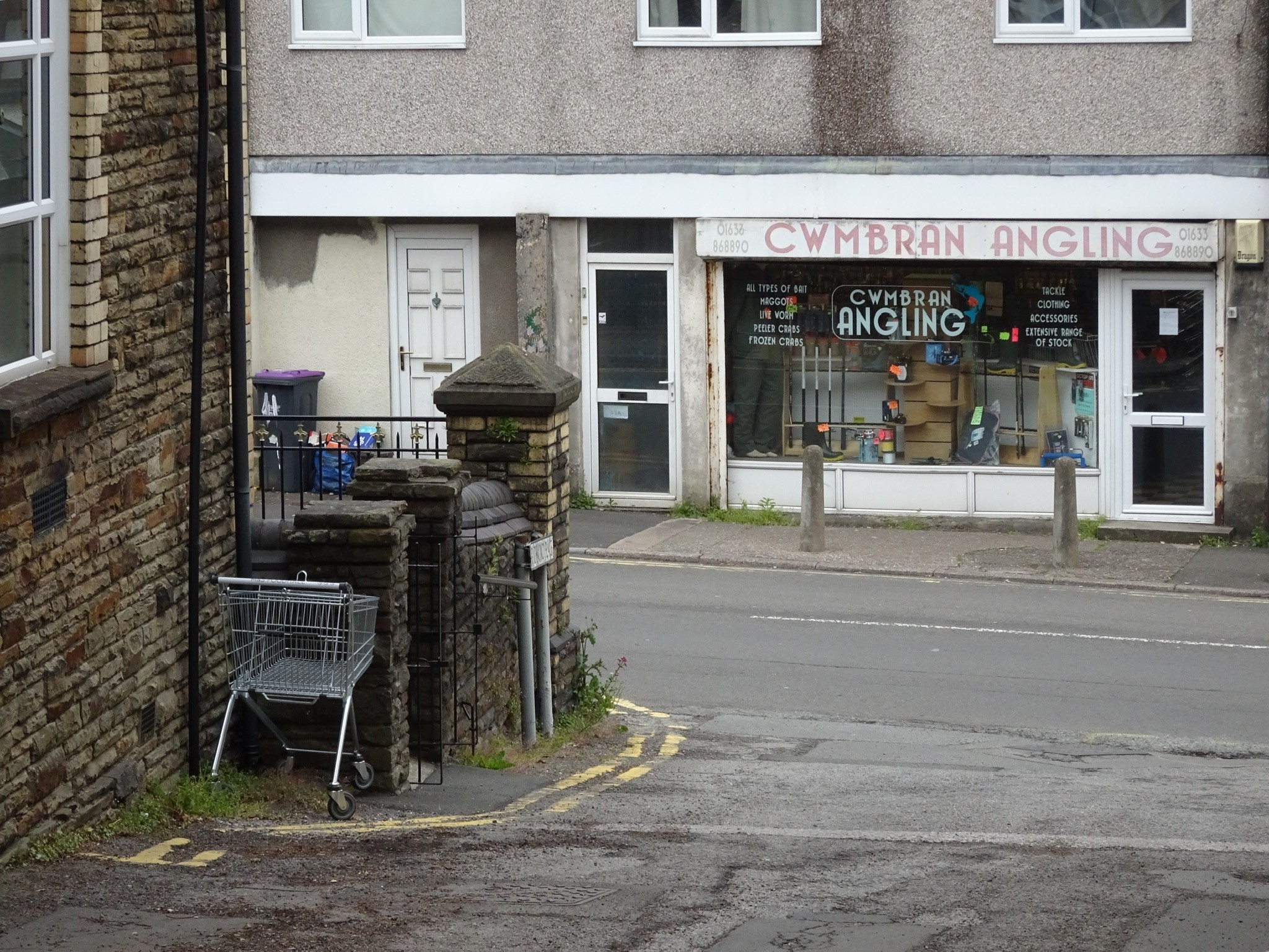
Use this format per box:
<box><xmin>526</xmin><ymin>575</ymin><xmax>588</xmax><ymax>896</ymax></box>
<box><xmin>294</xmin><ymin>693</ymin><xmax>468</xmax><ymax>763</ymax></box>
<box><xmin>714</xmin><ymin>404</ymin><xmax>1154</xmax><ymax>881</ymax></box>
<box><xmin>801</xmin><ymin>423</ymin><xmax>845</xmax><ymax>461</ymax></box>
<box><xmin>988</xmin><ymin>330</ymin><xmax>1017</xmax><ymax>375</ymax></box>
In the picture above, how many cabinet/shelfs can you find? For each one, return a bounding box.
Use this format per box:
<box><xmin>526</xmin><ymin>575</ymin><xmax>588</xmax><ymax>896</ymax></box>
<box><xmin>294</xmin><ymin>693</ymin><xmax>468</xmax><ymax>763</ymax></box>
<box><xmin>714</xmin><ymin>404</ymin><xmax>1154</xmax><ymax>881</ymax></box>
<box><xmin>782</xmin><ymin>284</ymin><xmax>967</xmax><ymax>468</ymax></box>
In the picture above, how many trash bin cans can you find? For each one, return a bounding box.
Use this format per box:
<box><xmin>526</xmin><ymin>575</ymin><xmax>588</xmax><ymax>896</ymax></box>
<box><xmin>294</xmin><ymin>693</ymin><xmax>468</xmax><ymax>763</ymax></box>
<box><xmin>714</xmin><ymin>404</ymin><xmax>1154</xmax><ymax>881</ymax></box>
<box><xmin>251</xmin><ymin>368</ymin><xmax>326</xmax><ymax>491</ymax></box>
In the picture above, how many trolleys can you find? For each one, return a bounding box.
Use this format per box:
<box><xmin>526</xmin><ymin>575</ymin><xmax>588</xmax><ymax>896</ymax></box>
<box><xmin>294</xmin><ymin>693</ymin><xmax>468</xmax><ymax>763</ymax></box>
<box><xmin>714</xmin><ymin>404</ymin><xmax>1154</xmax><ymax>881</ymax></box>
<box><xmin>206</xmin><ymin>573</ymin><xmax>381</xmax><ymax>821</ymax></box>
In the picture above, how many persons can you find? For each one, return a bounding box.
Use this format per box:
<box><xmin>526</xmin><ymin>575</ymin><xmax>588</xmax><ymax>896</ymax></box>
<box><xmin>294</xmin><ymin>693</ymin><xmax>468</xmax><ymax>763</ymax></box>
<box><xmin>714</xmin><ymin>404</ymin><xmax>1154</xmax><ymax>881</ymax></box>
<box><xmin>724</xmin><ymin>263</ymin><xmax>792</xmax><ymax>457</ymax></box>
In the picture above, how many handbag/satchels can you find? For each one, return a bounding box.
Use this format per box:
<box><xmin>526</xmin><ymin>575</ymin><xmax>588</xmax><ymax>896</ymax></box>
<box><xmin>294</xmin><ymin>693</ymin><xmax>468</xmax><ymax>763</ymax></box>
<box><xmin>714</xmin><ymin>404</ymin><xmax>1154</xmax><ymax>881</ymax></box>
<box><xmin>314</xmin><ymin>448</ymin><xmax>357</xmax><ymax>494</ymax></box>
